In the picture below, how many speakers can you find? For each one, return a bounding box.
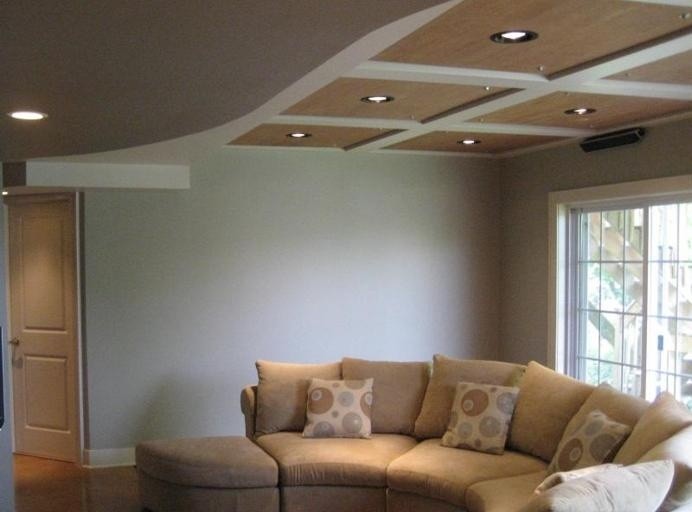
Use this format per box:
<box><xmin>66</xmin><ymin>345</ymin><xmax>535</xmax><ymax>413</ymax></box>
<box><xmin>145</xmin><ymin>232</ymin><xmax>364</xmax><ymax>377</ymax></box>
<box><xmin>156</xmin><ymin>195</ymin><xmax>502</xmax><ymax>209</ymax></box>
<box><xmin>580</xmin><ymin>127</ymin><xmax>644</xmax><ymax>152</ymax></box>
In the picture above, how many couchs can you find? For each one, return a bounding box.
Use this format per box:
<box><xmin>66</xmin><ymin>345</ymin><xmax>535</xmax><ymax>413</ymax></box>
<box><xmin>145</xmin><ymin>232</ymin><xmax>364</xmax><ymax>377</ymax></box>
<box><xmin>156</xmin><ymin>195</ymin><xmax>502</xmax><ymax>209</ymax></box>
<box><xmin>241</xmin><ymin>381</ymin><xmax>692</xmax><ymax>510</ymax></box>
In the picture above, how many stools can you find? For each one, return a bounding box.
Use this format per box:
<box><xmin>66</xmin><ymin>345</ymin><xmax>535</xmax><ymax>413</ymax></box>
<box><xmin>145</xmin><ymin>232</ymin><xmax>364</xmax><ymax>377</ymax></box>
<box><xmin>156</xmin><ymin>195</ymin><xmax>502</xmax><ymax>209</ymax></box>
<box><xmin>132</xmin><ymin>435</ymin><xmax>279</xmax><ymax>512</ymax></box>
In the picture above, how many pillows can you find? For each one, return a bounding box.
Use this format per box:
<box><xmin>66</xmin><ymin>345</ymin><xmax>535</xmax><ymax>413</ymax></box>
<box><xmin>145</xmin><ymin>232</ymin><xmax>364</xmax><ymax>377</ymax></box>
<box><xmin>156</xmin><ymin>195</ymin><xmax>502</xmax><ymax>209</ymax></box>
<box><xmin>523</xmin><ymin>456</ymin><xmax>676</xmax><ymax>511</ymax></box>
<box><xmin>510</xmin><ymin>359</ymin><xmax>596</xmax><ymax>468</ymax></box>
<box><xmin>301</xmin><ymin>376</ymin><xmax>376</xmax><ymax>441</ymax></box>
<box><xmin>612</xmin><ymin>388</ymin><xmax>688</xmax><ymax>468</ymax></box>
<box><xmin>550</xmin><ymin>381</ymin><xmax>653</xmax><ymax>480</ymax></box>
<box><xmin>255</xmin><ymin>357</ymin><xmax>345</xmax><ymax>435</ymax></box>
<box><xmin>413</xmin><ymin>352</ymin><xmax>527</xmax><ymax>451</ymax></box>
<box><xmin>338</xmin><ymin>354</ymin><xmax>432</xmax><ymax>437</ymax></box>
<box><xmin>548</xmin><ymin>407</ymin><xmax>631</xmax><ymax>474</ymax></box>
<box><xmin>438</xmin><ymin>381</ymin><xmax>521</xmax><ymax>456</ymax></box>
